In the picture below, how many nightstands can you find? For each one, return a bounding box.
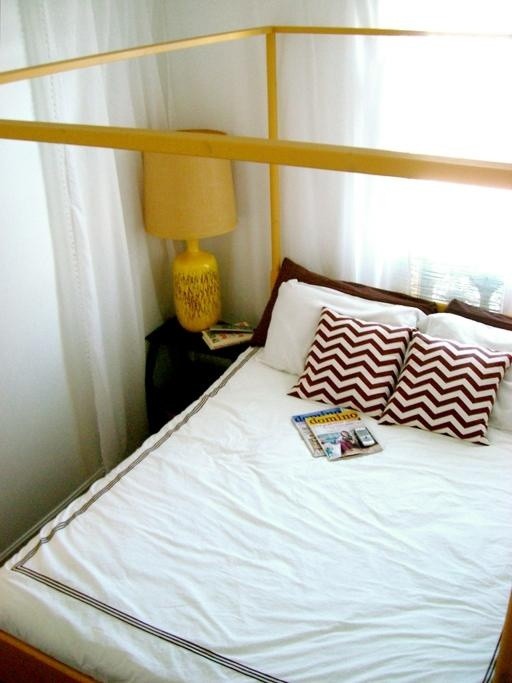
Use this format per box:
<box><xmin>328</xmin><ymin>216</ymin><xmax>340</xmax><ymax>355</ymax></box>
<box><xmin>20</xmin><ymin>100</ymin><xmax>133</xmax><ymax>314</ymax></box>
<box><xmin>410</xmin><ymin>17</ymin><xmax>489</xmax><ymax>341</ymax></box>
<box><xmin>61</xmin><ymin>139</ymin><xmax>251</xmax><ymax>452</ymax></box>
<box><xmin>141</xmin><ymin>310</ymin><xmax>257</xmax><ymax>441</ymax></box>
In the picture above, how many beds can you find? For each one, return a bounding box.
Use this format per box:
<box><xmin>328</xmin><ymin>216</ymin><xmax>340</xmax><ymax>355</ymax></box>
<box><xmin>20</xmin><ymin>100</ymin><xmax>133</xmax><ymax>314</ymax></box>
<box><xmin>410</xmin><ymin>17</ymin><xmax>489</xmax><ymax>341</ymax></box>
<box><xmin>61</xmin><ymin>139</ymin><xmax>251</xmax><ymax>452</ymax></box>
<box><xmin>1</xmin><ymin>254</ymin><xmax>512</xmax><ymax>682</ymax></box>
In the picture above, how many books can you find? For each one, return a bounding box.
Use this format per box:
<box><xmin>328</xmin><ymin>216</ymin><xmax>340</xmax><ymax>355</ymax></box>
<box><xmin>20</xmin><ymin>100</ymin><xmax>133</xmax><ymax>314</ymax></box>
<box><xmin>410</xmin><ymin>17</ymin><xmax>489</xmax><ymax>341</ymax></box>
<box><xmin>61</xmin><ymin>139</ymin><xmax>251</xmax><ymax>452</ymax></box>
<box><xmin>290</xmin><ymin>405</ymin><xmax>343</xmax><ymax>457</ymax></box>
<box><xmin>201</xmin><ymin>320</ymin><xmax>254</xmax><ymax>350</ymax></box>
<box><xmin>304</xmin><ymin>409</ymin><xmax>384</xmax><ymax>461</ymax></box>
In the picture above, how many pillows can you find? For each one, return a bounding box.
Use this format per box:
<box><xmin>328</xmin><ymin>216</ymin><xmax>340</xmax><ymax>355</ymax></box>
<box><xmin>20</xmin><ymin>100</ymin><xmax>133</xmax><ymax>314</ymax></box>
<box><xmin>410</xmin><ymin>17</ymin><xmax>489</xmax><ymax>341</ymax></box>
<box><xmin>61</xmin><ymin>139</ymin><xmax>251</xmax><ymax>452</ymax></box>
<box><xmin>257</xmin><ymin>276</ymin><xmax>429</xmax><ymax>381</ymax></box>
<box><xmin>442</xmin><ymin>295</ymin><xmax>511</xmax><ymax>333</ymax></box>
<box><xmin>373</xmin><ymin>323</ymin><xmax>511</xmax><ymax>447</ymax></box>
<box><xmin>285</xmin><ymin>303</ymin><xmax>420</xmax><ymax>421</ymax></box>
<box><xmin>421</xmin><ymin>309</ymin><xmax>511</xmax><ymax>435</ymax></box>
<box><xmin>248</xmin><ymin>254</ymin><xmax>440</xmax><ymax>350</ymax></box>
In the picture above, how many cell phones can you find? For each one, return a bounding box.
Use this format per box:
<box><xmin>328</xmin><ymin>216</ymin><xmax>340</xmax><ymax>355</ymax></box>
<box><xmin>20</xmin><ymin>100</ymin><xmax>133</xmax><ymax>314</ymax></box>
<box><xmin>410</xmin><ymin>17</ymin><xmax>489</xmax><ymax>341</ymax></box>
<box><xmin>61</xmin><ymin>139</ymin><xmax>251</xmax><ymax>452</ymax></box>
<box><xmin>354</xmin><ymin>427</ymin><xmax>375</xmax><ymax>447</ymax></box>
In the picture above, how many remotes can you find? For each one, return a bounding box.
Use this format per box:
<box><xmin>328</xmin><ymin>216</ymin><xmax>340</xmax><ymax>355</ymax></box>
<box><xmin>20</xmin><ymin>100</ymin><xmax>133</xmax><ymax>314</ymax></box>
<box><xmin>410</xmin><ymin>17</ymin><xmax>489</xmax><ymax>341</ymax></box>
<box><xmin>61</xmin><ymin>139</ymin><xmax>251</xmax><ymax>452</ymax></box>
<box><xmin>209</xmin><ymin>325</ymin><xmax>254</xmax><ymax>333</ymax></box>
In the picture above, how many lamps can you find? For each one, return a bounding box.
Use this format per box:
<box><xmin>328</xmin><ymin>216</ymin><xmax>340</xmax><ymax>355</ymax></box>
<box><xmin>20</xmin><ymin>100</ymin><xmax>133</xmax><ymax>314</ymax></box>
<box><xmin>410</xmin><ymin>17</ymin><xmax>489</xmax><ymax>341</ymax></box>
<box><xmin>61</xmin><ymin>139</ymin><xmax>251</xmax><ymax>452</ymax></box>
<box><xmin>137</xmin><ymin>123</ymin><xmax>240</xmax><ymax>339</ymax></box>
